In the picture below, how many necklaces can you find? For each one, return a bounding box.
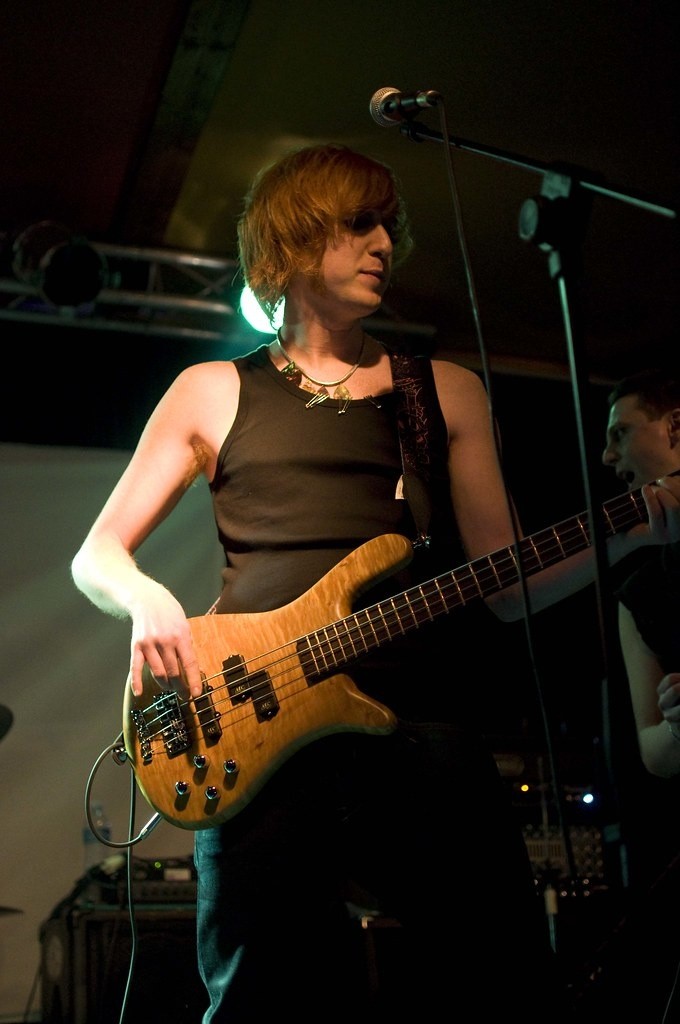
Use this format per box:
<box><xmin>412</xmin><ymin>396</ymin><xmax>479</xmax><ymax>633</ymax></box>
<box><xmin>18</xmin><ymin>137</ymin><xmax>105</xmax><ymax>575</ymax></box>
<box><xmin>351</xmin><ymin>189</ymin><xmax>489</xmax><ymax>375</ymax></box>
<box><xmin>274</xmin><ymin>328</ymin><xmax>371</xmax><ymax>416</ymax></box>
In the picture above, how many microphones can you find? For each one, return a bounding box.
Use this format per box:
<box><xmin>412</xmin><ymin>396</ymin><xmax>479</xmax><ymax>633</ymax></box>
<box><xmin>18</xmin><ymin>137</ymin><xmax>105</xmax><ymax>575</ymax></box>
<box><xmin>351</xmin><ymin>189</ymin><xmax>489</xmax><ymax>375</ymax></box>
<box><xmin>370</xmin><ymin>86</ymin><xmax>439</xmax><ymax>126</ymax></box>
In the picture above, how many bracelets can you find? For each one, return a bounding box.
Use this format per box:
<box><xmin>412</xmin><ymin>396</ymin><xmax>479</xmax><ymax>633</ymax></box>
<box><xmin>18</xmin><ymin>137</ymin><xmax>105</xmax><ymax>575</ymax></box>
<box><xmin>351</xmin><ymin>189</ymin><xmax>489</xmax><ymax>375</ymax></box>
<box><xmin>661</xmin><ymin>720</ymin><xmax>680</xmax><ymax>747</ymax></box>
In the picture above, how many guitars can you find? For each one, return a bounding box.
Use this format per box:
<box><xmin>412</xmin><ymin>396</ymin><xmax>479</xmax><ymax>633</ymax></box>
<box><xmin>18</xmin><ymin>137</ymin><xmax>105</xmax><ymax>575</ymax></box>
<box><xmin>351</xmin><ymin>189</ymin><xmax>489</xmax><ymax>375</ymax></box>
<box><xmin>122</xmin><ymin>471</ymin><xmax>680</xmax><ymax>830</ymax></box>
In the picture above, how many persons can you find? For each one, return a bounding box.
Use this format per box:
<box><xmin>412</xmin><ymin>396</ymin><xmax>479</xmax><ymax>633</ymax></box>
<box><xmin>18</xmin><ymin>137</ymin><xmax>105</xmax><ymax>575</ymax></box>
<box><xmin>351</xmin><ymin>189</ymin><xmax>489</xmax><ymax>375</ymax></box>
<box><xmin>595</xmin><ymin>378</ymin><xmax>680</xmax><ymax>782</ymax></box>
<box><xmin>67</xmin><ymin>140</ymin><xmax>680</xmax><ymax>1024</ymax></box>
